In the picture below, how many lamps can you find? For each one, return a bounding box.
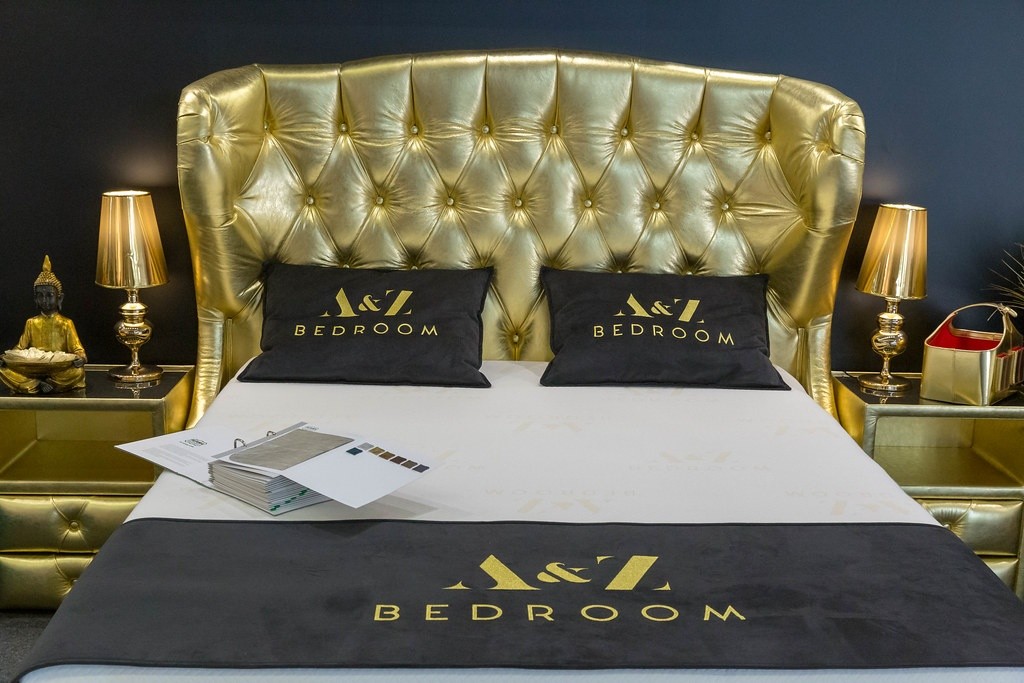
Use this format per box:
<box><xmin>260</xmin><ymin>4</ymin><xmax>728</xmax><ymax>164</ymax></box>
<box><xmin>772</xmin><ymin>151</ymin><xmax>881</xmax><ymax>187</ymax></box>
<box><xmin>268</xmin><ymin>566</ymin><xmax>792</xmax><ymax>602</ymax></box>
<box><xmin>854</xmin><ymin>202</ymin><xmax>928</xmax><ymax>393</ymax></box>
<box><xmin>95</xmin><ymin>190</ymin><xmax>170</xmax><ymax>383</ymax></box>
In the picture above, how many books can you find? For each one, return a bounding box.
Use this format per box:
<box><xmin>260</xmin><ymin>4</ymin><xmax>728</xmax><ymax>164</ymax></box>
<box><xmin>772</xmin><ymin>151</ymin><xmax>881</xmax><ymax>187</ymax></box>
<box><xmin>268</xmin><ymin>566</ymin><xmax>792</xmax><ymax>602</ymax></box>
<box><xmin>114</xmin><ymin>421</ymin><xmax>432</xmax><ymax>517</ymax></box>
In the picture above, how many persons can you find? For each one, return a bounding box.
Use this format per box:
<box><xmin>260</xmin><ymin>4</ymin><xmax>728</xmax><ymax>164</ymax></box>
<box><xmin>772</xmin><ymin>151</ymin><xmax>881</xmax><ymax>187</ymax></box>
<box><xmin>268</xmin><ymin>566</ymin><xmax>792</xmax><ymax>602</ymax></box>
<box><xmin>0</xmin><ymin>254</ymin><xmax>88</xmax><ymax>395</ymax></box>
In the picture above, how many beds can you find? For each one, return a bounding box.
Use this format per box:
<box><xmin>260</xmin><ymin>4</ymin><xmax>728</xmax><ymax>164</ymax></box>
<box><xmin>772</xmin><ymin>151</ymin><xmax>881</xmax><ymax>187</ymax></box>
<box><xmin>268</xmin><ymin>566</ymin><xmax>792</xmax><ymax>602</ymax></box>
<box><xmin>10</xmin><ymin>48</ymin><xmax>1024</xmax><ymax>683</ymax></box>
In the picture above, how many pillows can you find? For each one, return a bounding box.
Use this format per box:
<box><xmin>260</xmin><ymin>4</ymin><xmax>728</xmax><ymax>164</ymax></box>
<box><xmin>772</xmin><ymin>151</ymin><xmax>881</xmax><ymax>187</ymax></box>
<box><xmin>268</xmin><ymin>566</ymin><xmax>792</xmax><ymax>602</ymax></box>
<box><xmin>238</xmin><ymin>260</ymin><xmax>494</xmax><ymax>390</ymax></box>
<box><xmin>539</xmin><ymin>268</ymin><xmax>792</xmax><ymax>390</ymax></box>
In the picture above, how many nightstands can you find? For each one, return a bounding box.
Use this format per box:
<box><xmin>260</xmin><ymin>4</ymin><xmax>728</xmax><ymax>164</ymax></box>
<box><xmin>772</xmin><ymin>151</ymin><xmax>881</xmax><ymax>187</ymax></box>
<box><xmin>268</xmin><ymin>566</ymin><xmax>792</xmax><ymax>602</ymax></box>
<box><xmin>0</xmin><ymin>364</ymin><xmax>196</xmax><ymax>614</ymax></box>
<box><xmin>830</xmin><ymin>371</ymin><xmax>1024</xmax><ymax>600</ymax></box>
